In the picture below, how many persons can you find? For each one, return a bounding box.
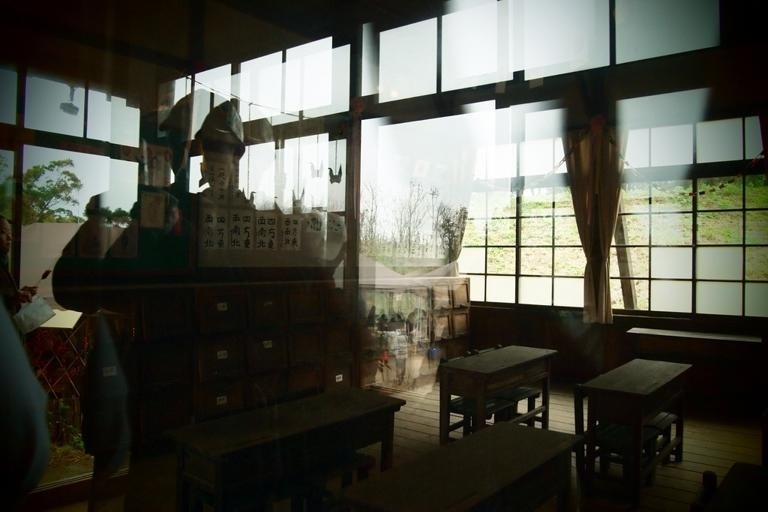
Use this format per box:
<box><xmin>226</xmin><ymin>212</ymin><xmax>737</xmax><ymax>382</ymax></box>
<box><xmin>0</xmin><ymin>215</ymin><xmax>38</xmax><ymax>328</ymax></box>
<box><xmin>49</xmin><ymin>88</ymin><xmax>263</xmax><ymax>510</ymax></box>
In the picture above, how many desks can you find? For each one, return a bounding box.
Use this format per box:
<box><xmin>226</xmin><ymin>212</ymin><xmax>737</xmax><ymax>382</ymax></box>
<box><xmin>704</xmin><ymin>462</ymin><xmax>768</xmax><ymax>509</ymax></box>
<box><xmin>327</xmin><ymin>423</ymin><xmax>583</xmax><ymax>509</ymax></box>
<box><xmin>173</xmin><ymin>385</ymin><xmax>407</xmax><ymax>509</ymax></box>
<box><xmin>573</xmin><ymin>357</ymin><xmax>693</xmax><ymax>500</ymax></box>
<box><xmin>436</xmin><ymin>345</ymin><xmax>560</xmax><ymax>447</ymax></box>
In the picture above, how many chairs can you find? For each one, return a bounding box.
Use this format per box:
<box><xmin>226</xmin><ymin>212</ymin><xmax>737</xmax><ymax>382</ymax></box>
<box><xmin>440</xmin><ymin>351</ymin><xmax>516</xmax><ymax>443</ymax></box>
<box><xmin>475</xmin><ymin>345</ymin><xmax>542</xmax><ymax>428</ymax></box>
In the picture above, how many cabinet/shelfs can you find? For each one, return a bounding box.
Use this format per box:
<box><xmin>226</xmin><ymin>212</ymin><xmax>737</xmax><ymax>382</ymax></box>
<box><xmin>92</xmin><ymin>278</ymin><xmax>471</xmax><ymax>452</ymax></box>
